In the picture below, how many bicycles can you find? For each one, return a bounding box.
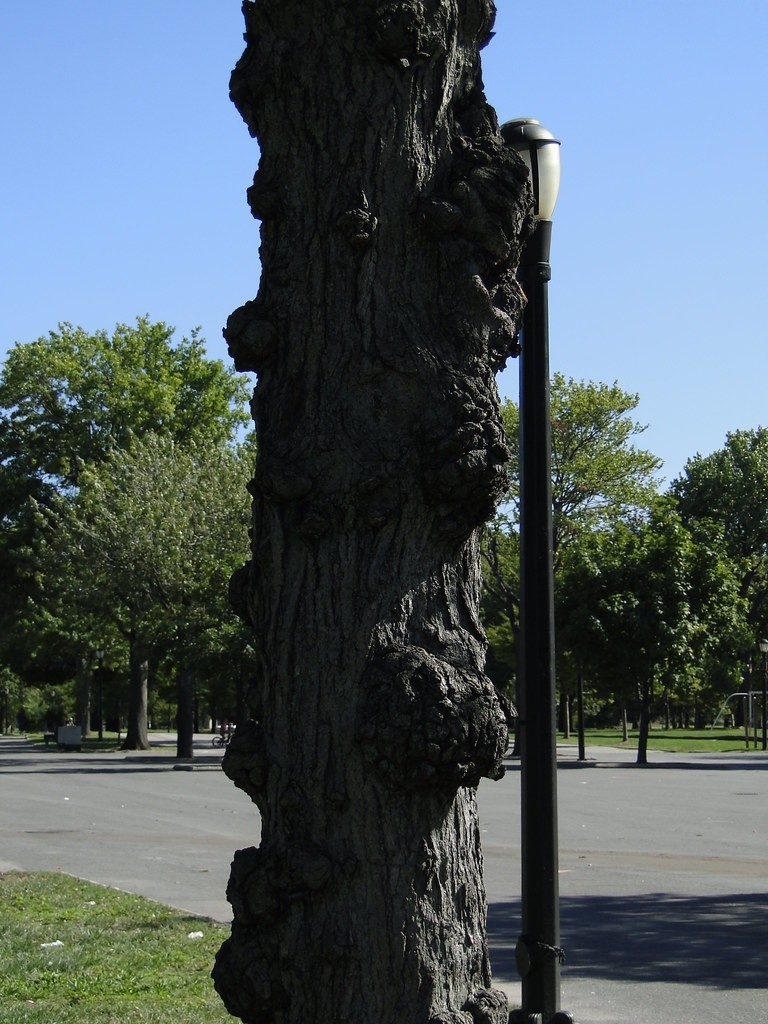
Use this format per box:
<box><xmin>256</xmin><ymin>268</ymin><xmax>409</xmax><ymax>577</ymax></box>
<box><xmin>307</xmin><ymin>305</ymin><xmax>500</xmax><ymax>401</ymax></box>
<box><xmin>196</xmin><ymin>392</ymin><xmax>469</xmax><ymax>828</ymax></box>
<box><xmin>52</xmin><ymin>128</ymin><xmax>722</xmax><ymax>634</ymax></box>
<box><xmin>211</xmin><ymin>731</ymin><xmax>234</xmax><ymax>749</ymax></box>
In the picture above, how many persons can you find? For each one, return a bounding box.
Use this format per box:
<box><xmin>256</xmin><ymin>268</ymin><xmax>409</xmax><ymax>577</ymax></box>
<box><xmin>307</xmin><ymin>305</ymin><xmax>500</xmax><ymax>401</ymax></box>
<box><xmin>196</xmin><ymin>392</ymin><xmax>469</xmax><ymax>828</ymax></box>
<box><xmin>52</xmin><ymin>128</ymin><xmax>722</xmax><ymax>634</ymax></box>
<box><xmin>219</xmin><ymin>718</ymin><xmax>230</xmax><ymax>743</ymax></box>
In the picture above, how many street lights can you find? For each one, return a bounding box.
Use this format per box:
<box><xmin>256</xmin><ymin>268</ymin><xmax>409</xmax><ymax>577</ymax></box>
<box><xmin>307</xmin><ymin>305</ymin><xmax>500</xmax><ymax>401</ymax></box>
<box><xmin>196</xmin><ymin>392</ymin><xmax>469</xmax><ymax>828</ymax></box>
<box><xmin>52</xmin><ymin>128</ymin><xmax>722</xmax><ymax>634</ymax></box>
<box><xmin>476</xmin><ymin>115</ymin><xmax>578</xmax><ymax>1023</ymax></box>
<box><xmin>757</xmin><ymin>638</ymin><xmax>768</xmax><ymax>751</ymax></box>
